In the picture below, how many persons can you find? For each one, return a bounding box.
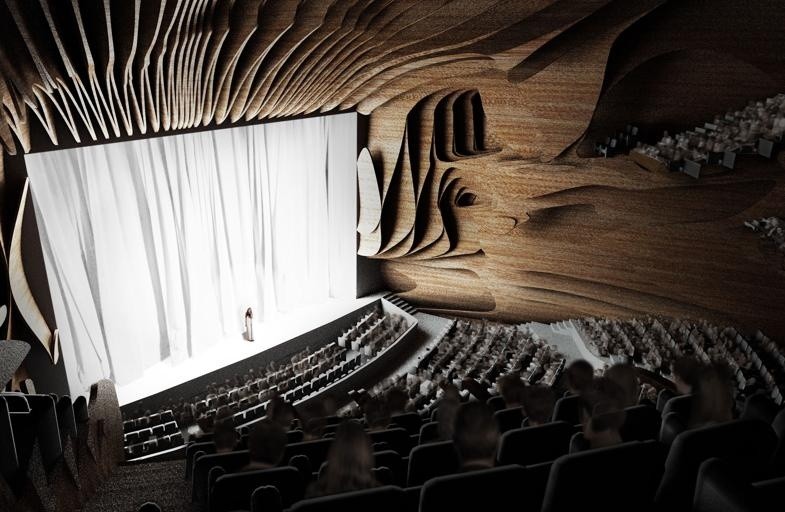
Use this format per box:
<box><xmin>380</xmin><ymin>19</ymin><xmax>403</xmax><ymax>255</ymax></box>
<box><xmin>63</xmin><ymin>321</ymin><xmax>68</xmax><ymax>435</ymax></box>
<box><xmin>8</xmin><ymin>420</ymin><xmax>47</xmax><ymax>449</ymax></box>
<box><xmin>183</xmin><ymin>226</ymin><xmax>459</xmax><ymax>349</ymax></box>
<box><xmin>630</xmin><ymin>91</ymin><xmax>784</xmax><ymax>166</ymax></box>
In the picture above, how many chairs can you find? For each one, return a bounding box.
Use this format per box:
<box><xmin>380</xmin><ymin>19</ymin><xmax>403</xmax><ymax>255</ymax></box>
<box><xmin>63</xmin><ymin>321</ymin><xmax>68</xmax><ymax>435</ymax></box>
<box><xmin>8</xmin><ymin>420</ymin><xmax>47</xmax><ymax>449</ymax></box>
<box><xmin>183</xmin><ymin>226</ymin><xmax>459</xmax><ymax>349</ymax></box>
<box><xmin>122</xmin><ymin>312</ymin><xmax>785</xmax><ymax>512</ymax></box>
<box><xmin>15</xmin><ymin>380</ymin><xmax>88</xmax><ymax>512</ymax></box>
<box><xmin>591</xmin><ymin>91</ymin><xmax>785</xmax><ymax>255</ymax></box>
